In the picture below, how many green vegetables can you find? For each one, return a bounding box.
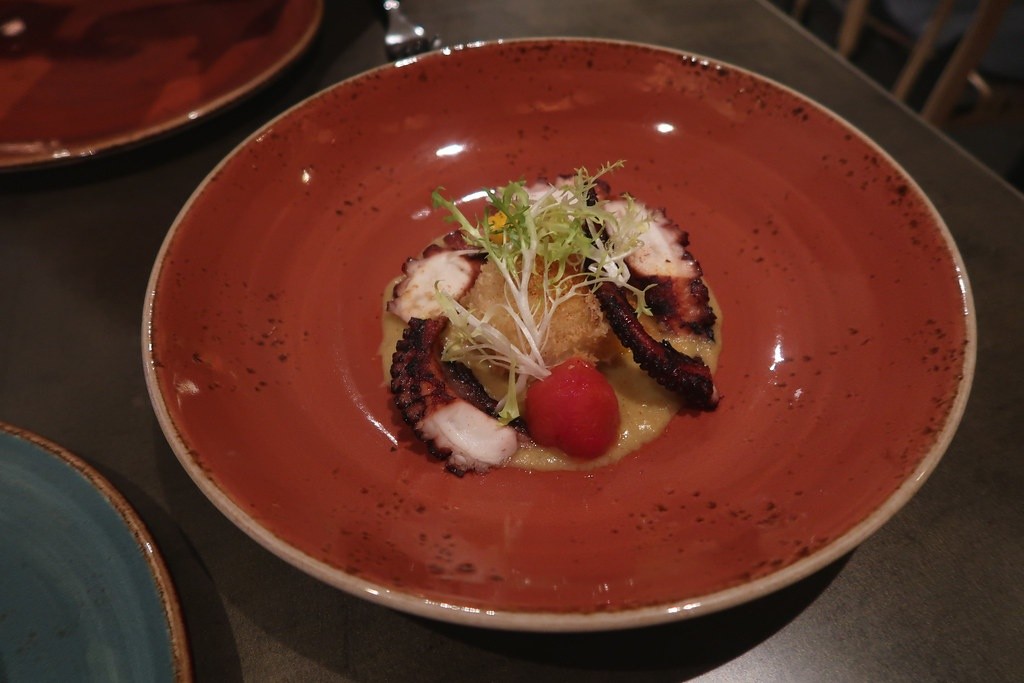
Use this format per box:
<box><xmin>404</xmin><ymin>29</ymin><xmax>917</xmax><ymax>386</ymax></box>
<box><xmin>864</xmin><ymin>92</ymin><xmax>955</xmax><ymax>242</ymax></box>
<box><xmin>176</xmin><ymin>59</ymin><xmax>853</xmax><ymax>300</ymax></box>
<box><xmin>430</xmin><ymin>157</ymin><xmax>660</xmax><ymax>424</ymax></box>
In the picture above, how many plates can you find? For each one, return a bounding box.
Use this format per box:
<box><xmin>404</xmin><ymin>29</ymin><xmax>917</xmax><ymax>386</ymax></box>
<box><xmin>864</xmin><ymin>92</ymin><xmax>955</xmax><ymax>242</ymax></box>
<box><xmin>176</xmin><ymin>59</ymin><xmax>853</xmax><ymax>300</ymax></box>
<box><xmin>142</xmin><ymin>34</ymin><xmax>978</xmax><ymax>630</ymax></box>
<box><xmin>0</xmin><ymin>0</ymin><xmax>325</xmax><ymax>168</ymax></box>
<box><xmin>0</xmin><ymin>422</ymin><xmax>190</xmax><ymax>683</ymax></box>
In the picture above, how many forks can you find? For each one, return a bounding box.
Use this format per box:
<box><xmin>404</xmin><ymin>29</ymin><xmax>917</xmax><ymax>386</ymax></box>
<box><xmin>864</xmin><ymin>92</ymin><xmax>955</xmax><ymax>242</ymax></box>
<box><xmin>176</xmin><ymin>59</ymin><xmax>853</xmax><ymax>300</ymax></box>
<box><xmin>379</xmin><ymin>0</ymin><xmax>443</xmax><ymax>58</ymax></box>
